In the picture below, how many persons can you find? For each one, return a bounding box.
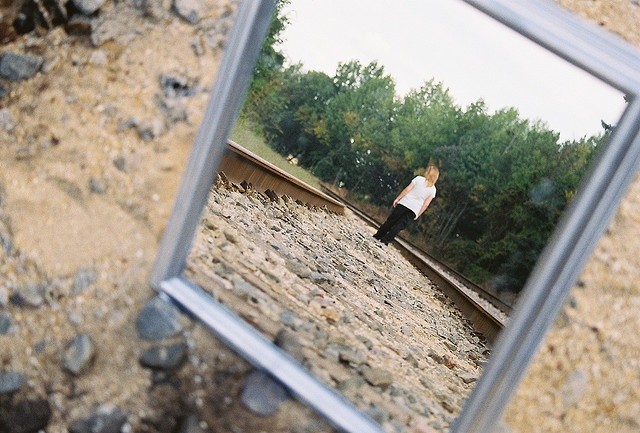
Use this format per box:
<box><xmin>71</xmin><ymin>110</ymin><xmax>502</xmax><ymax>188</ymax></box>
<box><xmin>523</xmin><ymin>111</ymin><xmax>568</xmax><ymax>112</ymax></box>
<box><xmin>373</xmin><ymin>165</ymin><xmax>440</xmax><ymax>246</ymax></box>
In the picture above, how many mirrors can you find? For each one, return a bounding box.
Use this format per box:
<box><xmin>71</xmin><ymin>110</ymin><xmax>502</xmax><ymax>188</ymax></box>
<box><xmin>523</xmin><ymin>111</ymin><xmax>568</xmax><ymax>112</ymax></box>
<box><xmin>150</xmin><ymin>1</ymin><xmax>640</xmax><ymax>432</ymax></box>
